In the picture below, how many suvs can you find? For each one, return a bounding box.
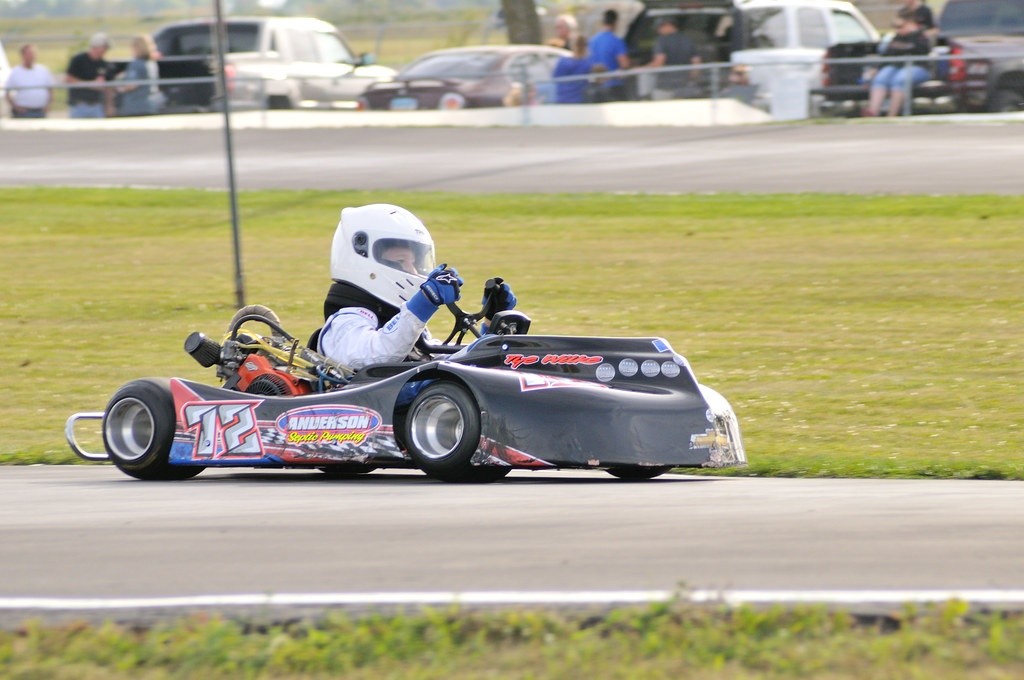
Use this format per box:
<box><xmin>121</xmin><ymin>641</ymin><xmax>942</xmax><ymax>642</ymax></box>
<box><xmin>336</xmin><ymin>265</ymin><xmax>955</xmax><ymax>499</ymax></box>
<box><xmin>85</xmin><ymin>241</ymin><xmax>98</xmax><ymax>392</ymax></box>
<box><xmin>150</xmin><ymin>17</ymin><xmax>397</xmax><ymax>112</ymax></box>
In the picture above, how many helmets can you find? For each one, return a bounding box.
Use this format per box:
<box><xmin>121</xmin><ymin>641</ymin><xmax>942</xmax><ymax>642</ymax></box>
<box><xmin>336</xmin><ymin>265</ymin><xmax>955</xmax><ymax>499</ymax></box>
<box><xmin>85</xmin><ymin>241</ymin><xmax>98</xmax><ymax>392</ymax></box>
<box><xmin>330</xmin><ymin>203</ymin><xmax>436</xmax><ymax>310</ymax></box>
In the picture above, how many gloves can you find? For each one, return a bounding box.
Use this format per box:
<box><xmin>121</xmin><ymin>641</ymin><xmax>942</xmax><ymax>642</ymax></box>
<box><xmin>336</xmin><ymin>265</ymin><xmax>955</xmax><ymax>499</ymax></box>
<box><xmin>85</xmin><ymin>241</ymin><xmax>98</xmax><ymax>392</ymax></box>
<box><xmin>419</xmin><ymin>263</ymin><xmax>463</xmax><ymax>305</ymax></box>
<box><xmin>482</xmin><ymin>278</ymin><xmax>517</xmax><ymax>321</ymax></box>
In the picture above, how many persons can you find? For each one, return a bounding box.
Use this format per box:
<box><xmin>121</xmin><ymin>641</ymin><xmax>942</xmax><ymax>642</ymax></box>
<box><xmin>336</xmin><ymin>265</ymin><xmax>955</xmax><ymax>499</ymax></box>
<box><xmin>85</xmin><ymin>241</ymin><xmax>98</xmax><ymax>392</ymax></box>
<box><xmin>589</xmin><ymin>9</ymin><xmax>640</xmax><ymax>102</ymax></box>
<box><xmin>555</xmin><ymin>13</ymin><xmax>579</xmax><ymax>51</ymax></box>
<box><xmin>863</xmin><ymin>0</ymin><xmax>940</xmax><ymax>116</ymax></box>
<box><xmin>5</xmin><ymin>44</ymin><xmax>52</xmax><ymax>119</ymax></box>
<box><xmin>583</xmin><ymin>64</ymin><xmax>613</xmax><ymax>104</ymax></box>
<box><xmin>553</xmin><ymin>34</ymin><xmax>595</xmax><ymax>104</ymax></box>
<box><xmin>62</xmin><ymin>32</ymin><xmax>116</xmax><ymax>118</ymax></box>
<box><xmin>114</xmin><ymin>33</ymin><xmax>168</xmax><ymax>117</ymax></box>
<box><xmin>647</xmin><ymin>15</ymin><xmax>703</xmax><ymax>100</ymax></box>
<box><xmin>317</xmin><ymin>203</ymin><xmax>517</xmax><ymax>405</ymax></box>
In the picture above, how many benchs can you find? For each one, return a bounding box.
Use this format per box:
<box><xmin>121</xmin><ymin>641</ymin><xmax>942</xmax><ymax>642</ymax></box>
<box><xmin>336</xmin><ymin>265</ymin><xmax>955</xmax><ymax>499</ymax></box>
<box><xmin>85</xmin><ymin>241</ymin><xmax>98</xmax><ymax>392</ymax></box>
<box><xmin>809</xmin><ymin>37</ymin><xmax>954</xmax><ymax>116</ymax></box>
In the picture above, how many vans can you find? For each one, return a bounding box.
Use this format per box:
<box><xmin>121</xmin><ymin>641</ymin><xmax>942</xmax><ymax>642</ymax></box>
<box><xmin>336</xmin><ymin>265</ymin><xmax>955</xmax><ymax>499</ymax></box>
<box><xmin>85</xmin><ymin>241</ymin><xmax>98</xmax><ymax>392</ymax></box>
<box><xmin>622</xmin><ymin>0</ymin><xmax>881</xmax><ymax>118</ymax></box>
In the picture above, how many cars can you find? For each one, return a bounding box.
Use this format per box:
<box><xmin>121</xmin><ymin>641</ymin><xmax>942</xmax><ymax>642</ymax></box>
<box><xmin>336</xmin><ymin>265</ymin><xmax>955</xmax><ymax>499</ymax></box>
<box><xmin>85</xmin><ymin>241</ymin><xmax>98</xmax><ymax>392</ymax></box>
<box><xmin>362</xmin><ymin>47</ymin><xmax>575</xmax><ymax>110</ymax></box>
<box><xmin>945</xmin><ymin>35</ymin><xmax>1024</xmax><ymax>113</ymax></box>
